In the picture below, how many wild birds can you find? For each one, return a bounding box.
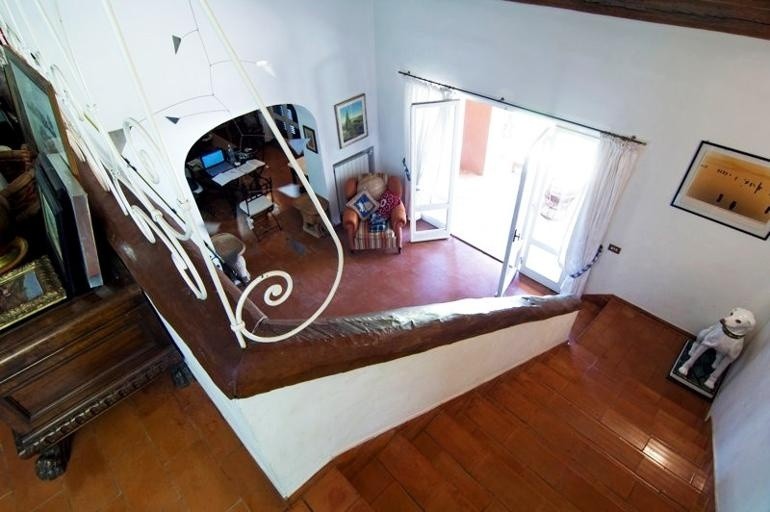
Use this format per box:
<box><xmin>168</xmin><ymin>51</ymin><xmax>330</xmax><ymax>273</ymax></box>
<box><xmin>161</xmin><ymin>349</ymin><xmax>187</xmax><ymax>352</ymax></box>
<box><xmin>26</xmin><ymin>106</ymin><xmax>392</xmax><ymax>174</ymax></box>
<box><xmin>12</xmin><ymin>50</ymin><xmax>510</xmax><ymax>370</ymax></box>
<box><xmin>200</xmin><ymin>149</ymin><xmax>233</xmax><ymax>177</ymax></box>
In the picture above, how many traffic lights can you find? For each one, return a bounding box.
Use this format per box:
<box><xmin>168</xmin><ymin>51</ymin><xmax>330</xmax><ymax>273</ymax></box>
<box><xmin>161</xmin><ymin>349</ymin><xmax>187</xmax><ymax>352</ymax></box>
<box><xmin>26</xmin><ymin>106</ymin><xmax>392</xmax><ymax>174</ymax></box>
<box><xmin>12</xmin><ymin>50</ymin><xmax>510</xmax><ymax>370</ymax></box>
<box><xmin>345</xmin><ymin>176</ymin><xmax>401</xmax><ymax>221</ymax></box>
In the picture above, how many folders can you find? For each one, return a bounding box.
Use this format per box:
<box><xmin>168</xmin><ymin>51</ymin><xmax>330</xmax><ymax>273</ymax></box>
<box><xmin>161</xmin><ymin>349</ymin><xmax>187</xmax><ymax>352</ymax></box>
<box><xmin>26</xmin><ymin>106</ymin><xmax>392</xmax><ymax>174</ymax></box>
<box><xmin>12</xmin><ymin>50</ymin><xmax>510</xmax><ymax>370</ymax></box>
<box><xmin>334</xmin><ymin>153</ymin><xmax>370</xmax><ymax>216</ymax></box>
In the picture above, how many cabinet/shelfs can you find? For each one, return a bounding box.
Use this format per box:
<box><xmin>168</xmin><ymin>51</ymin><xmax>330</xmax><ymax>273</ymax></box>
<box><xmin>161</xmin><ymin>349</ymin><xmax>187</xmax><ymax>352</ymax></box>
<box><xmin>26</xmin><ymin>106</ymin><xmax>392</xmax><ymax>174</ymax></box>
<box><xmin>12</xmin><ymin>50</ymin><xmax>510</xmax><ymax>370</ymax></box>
<box><xmin>227</xmin><ymin>143</ymin><xmax>237</xmax><ymax>165</ymax></box>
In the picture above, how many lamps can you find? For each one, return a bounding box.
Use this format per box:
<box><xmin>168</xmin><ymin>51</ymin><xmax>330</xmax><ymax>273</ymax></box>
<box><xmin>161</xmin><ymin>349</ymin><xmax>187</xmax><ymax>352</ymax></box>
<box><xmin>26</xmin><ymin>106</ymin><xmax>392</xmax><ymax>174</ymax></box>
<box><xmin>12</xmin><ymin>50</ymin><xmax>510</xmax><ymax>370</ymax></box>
<box><xmin>240</xmin><ymin>135</ymin><xmax>268</xmax><ymax>187</ymax></box>
<box><xmin>342</xmin><ymin>177</ymin><xmax>407</xmax><ymax>254</ymax></box>
<box><xmin>239</xmin><ymin>176</ymin><xmax>281</xmax><ymax>242</ymax></box>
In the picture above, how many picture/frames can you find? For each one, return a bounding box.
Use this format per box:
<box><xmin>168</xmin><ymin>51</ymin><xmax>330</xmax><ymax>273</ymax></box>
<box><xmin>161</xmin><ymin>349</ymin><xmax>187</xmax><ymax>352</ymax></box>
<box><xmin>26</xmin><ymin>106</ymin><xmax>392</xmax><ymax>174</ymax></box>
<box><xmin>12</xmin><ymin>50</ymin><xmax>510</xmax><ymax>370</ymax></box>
<box><xmin>287</xmin><ymin>157</ymin><xmax>309</xmax><ymax>193</ymax></box>
<box><xmin>186</xmin><ymin>132</ymin><xmax>265</xmax><ymax>218</ymax></box>
<box><xmin>0</xmin><ymin>275</ymin><xmax>190</xmax><ymax>481</ymax></box>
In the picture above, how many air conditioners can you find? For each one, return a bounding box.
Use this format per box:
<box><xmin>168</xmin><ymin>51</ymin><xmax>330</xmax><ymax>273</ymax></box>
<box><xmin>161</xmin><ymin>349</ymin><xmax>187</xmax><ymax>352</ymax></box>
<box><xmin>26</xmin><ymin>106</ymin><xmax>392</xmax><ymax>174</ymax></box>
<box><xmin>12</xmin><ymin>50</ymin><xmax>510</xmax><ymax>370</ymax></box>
<box><xmin>679</xmin><ymin>308</ymin><xmax>756</xmax><ymax>389</ymax></box>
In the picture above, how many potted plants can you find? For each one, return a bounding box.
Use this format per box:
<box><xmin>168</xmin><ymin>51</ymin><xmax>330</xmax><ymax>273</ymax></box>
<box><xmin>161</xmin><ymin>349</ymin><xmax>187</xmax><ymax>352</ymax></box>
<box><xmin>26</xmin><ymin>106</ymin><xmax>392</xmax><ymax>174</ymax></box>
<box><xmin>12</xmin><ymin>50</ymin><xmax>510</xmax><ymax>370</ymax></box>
<box><xmin>334</xmin><ymin>93</ymin><xmax>368</xmax><ymax>149</ymax></box>
<box><xmin>0</xmin><ymin>45</ymin><xmax>104</xmax><ymax>331</ymax></box>
<box><xmin>302</xmin><ymin>125</ymin><xmax>319</xmax><ymax>155</ymax></box>
<box><xmin>670</xmin><ymin>140</ymin><xmax>770</xmax><ymax>241</ymax></box>
